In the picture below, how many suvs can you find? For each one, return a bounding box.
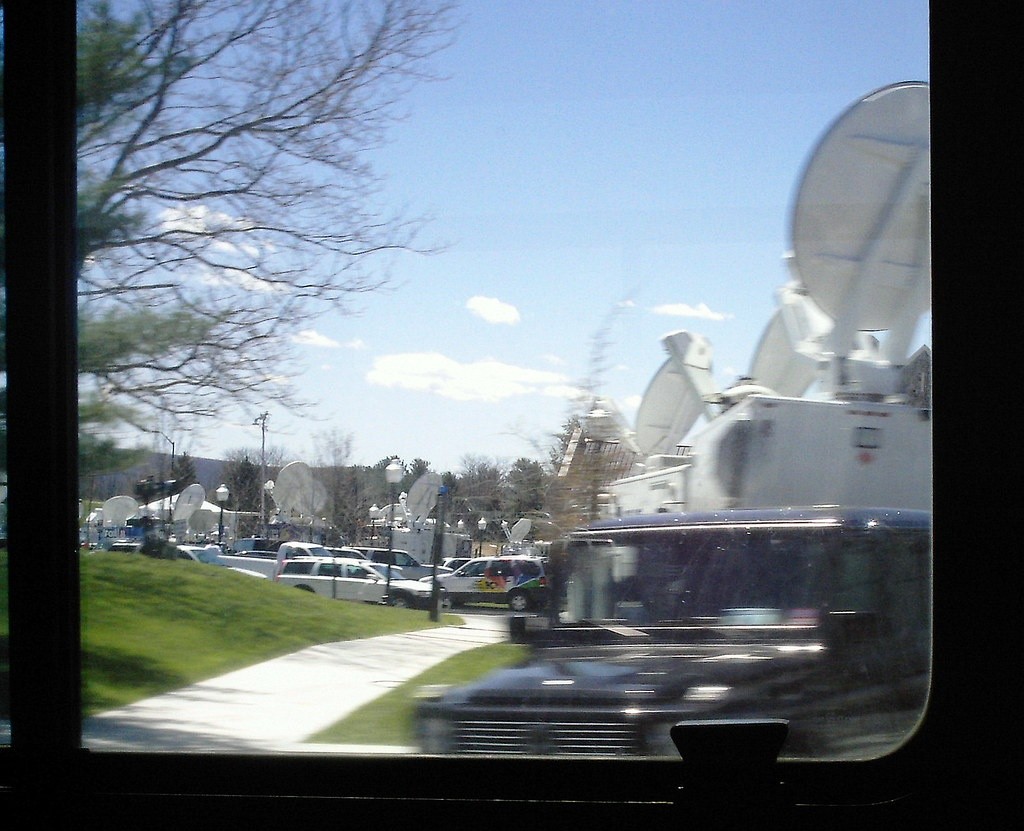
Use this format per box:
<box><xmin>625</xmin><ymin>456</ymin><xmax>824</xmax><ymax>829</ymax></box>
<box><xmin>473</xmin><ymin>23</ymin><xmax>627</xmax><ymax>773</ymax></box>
<box><xmin>275</xmin><ymin>542</ymin><xmax>480</xmax><ymax>611</ymax></box>
<box><xmin>419</xmin><ymin>556</ymin><xmax>549</xmax><ymax>611</ymax></box>
<box><xmin>416</xmin><ymin>506</ymin><xmax>932</xmax><ymax>753</ymax></box>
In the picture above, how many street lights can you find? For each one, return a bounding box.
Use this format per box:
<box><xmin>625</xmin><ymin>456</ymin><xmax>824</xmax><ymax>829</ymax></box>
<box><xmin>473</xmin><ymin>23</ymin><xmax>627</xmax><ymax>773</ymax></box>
<box><xmin>369</xmin><ymin>504</ymin><xmax>379</xmax><ymax>547</ymax></box>
<box><xmin>477</xmin><ymin>517</ymin><xmax>487</xmax><ymax>557</ymax></box>
<box><xmin>216</xmin><ymin>484</ymin><xmax>229</xmax><ymax>543</ymax></box>
